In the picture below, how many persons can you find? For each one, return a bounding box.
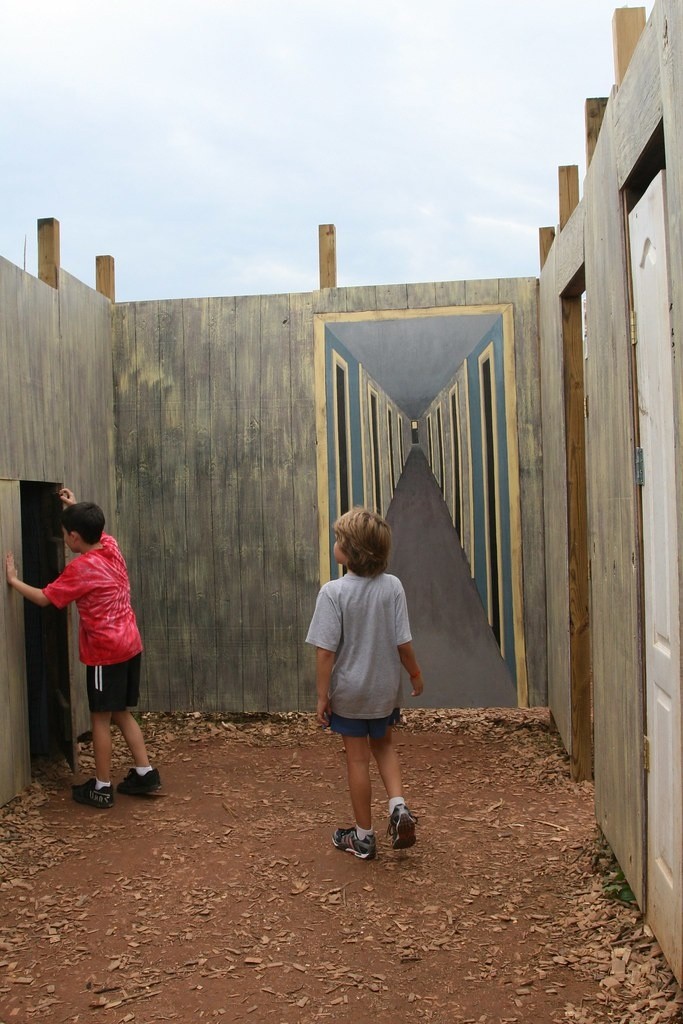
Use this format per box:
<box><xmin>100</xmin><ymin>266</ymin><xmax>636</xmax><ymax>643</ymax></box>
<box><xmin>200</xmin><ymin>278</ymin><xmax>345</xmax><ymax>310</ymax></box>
<box><xmin>6</xmin><ymin>488</ymin><xmax>162</xmax><ymax>808</ymax></box>
<box><xmin>304</xmin><ymin>510</ymin><xmax>424</xmax><ymax>860</ymax></box>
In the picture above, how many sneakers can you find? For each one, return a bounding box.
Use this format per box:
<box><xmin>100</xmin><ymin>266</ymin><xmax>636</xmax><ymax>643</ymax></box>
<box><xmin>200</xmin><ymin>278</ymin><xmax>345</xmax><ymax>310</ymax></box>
<box><xmin>117</xmin><ymin>767</ymin><xmax>162</xmax><ymax>794</ymax></box>
<box><xmin>71</xmin><ymin>777</ymin><xmax>114</xmax><ymax>809</ymax></box>
<box><xmin>332</xmin><ymin>828</ymin><xmax>376</xmax><ymax>860</ymax></box>
<box><xmin>385</xmin><ymin>804</ymin><xmax>418</xmax><ymax>849</ymax></box>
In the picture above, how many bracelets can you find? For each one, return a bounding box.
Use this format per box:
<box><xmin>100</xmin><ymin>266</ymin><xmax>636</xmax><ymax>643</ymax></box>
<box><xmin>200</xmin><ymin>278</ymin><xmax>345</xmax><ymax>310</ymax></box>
<box><xmin>410</xmin><ymin>670</ymin><xmax>420</xmax><ymax>678</ymax></box>
